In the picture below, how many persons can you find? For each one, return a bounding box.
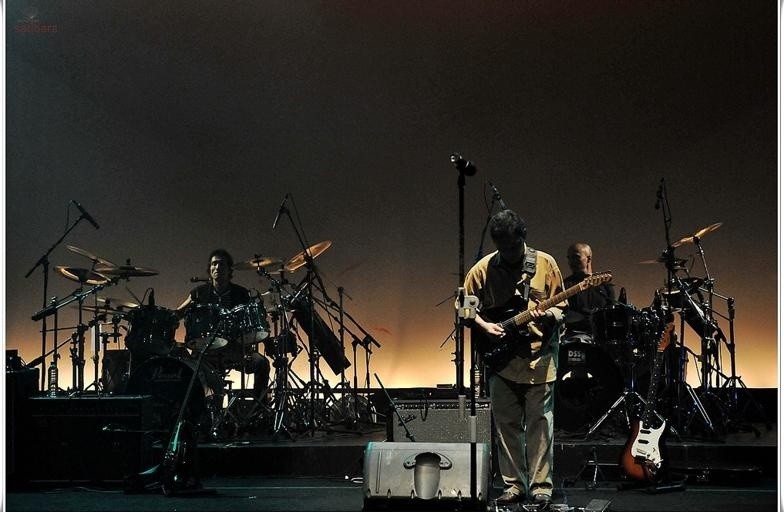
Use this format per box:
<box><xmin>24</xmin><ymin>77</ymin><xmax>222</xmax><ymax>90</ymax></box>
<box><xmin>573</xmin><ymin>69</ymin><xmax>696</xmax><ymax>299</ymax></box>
<box><xmin>171</xmin><ymin>247</ymin><xmax>270</xmax><ymax>406</ymax></box>
<box><xmin>563</xmin><ymin>242</ymin><xmax>615</xmax><ymax>332</ymax></box>
<box><xmin>455</xmin><ymin>210</ymin><xmax>568</xmax><ymax>505</ymax></box>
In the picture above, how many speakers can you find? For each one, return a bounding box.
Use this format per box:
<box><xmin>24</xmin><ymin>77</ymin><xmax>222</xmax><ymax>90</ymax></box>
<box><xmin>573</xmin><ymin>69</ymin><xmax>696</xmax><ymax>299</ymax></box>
<box><xmin>386</xmin><ymin>399</ymin><xmax>492</xmax><ymax>441</ymax></box>
<box><xmin>363</xmin><ymin>441</ymin><xmax>490</xmax><ymax>512</ymax></box>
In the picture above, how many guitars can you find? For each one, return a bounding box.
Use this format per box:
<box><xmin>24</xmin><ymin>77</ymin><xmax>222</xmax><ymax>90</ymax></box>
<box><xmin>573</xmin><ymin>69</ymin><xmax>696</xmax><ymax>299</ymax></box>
<box><xmin>620</xmin><ymin>321</ymin><xmax>675</xmax><ymax>479</ymax></box>
<box><xmin>474</xmin><ymin>269</ymin><xmax>614</xmax><ymax>361</ymax></box>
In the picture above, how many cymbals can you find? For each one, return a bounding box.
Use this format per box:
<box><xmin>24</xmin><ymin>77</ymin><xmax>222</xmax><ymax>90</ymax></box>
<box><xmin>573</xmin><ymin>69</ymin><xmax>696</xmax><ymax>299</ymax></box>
<box><xmin>231</xmin><ymin>255</ymin><xmax>282</xmax><ymax>271</ymax></box>
<box><xmin>53</xmin><ymin>264</ymin><xmax>111</xmax><ymax>285</ymax></box>
<box><xmin>70</xmin><ymin>305</ymin><xmax>128</xmax><ymax>317</ymax></box>
<box><xmin>94</xmin><ymin>295</ymin><xmax>139</xmax><ymax>308</ymax></box>
<box><xmin>95</xmin><ymin>264</ymin><xmax>159</xmax><ymax>276</ymax></box>
<box><xmin>66</xmin><ymin>244</ymin><xmax>117</xmax><ymax>268</ymax></box>
<box><xmin>284</xmin><ymin>238</ymin><xmax>333</xmax><ymax>270</ymax></box>
<box><xmin>672</xmin><ymin>277</ymin><xmax>703</xmax><ymax>289</ymax></box>
<box><xmin>673</xmin><ymin>222</ymin><xmax>722</xmax><ymax>247</ymax></box>
<box><xmin>641</xmin><ymin>255</ymin><xmax>682</xmax><ymax>263</ymax></box>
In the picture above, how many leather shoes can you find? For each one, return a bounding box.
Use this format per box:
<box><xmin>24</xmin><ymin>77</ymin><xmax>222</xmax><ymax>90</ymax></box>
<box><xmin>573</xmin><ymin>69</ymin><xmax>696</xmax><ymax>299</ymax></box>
<box><xmin>494</xmin><ymin>491</ymin><xmax>526</xmax><ymax>504</ymax></box>
<box><xmin>533</xmin><ymin>493</ymin><xmax>554</xmax><ymax>504</ymax></box>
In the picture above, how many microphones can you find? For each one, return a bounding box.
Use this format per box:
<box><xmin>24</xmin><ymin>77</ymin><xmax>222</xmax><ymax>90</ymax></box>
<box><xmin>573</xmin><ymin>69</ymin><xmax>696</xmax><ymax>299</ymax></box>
<box><xmin>76</xmin><ymin>203</ymin><xmax>100</xmax><ymax>230</ymax></box>
<box><xmin>654</xmin><ymin>183</ymin><xmax>663</xmax><ymax>210</ymax></box>
<box><xmin>272</xmin><ymin>198</ymin><xmax>288</xmax><ymax>229</ymax></box>
<box><xmin>489</xmin><ymin>181</ymin><xmax>508</xmax><ymax>210</ymax></box>
<box><xmin>447</xmin><ymin>152</ymin><xmax>478</xmax><ymax>177</ymax></box>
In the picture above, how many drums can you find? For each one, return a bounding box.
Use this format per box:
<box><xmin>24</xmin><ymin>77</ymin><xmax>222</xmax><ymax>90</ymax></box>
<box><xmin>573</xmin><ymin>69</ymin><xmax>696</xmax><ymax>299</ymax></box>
<box><xmin>128</xmin><ymin>353</ymin><xmax>208</xmax><ymax>413</ymax></box>
<box><xmin>184</xmin><ymin>303</ymin><xmax>233</xmax><ymax>351</ymax></box>
<box><xmin>264</xmin><ymin>335</ymin><xmax>296</xmax><ymax>356</ymax></box>
<box><xmin>124</xmin><ymin>304</ymin><xmax>179</xmax><ymax>354</ymax></box>
<box><xmin>232</xmin><ymin>302</ymin><xmax>272</xmax><ymax>346</ymax></box>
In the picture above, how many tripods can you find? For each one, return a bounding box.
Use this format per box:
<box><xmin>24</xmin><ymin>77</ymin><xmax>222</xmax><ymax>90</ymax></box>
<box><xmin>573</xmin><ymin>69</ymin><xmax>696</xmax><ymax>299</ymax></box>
<box><xmin>583</xmin><ymin>217</ymin><xmax>748</xmax><ymax>440</ymax></box>
<box><xmin>25</xmin><ymin>256</ymin><xmax>132</xmax><ymax>398</ymax></box>
<box><xmin>209</xmin><ymin>255</ymin><xmax>387</xmax><ymax>442</ymax></box>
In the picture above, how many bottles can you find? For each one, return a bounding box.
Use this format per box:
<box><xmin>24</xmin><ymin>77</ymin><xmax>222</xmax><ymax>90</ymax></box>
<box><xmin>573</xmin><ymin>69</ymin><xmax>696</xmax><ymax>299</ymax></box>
<box><xmin>47</xmin><ymin>361</ymin><xmax>58</xmax><ymax>396</ymax></box>
